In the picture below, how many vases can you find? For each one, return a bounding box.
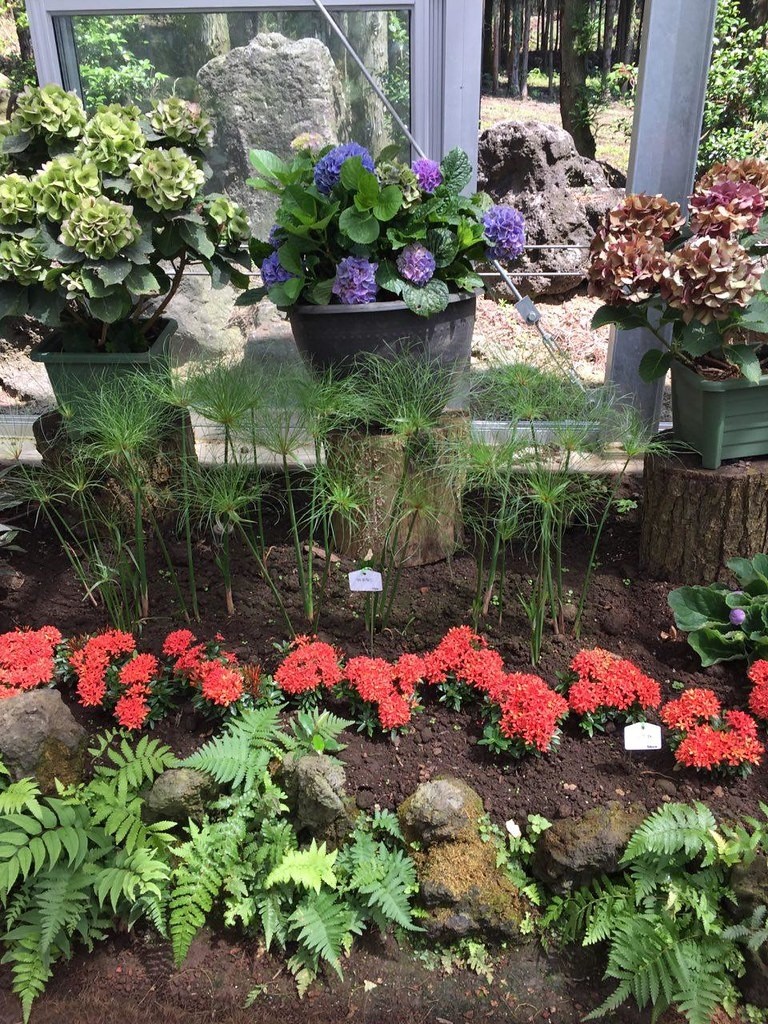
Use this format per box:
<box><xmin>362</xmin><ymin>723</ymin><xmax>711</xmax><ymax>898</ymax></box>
<box><xmin>31</xmin><ymin>317</ymin><xmax>179</xmax><ymax>419</ymax></box>
<box><xmin>670</xmin><ymin>360</ymin><xmax>768</xmax><ymax>470</ymax></box>
<box><xmin>272</xmin><ymin>287</ymin><xmax>487</xmax><ymax>434</ymax></box>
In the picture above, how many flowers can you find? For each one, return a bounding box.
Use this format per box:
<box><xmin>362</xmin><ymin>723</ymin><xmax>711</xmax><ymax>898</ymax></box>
<box><xmin>587</xmin><ymin>155</ymin><xmax>768</xmax><ymax>384</ymax></box>
<box><xmin>0</xmin><ymin>85</ymin><xmax>254</xmax><ymax>352</ymax></box>
<box><xmin>231</xmin><ymin>129</ymin><xmax>530</xmax><ymax>318</ymax></box>
